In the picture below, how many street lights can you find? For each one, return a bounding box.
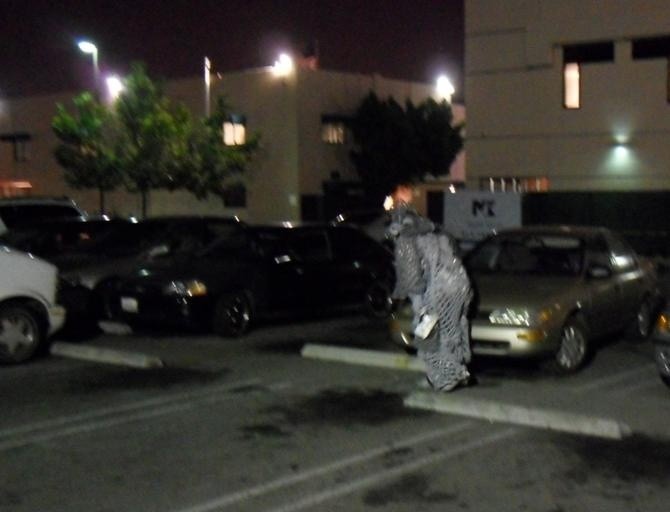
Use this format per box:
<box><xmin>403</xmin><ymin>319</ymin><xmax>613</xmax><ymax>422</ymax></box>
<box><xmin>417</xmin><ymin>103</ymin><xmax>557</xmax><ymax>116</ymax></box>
<box><xmin>76</xmin><ymin>40</ymin><xmax>104</xmax><ymax>213</ymax></box>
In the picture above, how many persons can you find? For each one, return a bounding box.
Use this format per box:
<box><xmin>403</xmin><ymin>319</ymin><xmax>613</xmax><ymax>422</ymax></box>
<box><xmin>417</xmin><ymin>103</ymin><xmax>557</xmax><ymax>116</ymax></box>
<box><xmin>382</xmin><ymin>186</ymin><xmax>475</xmax><ymax>393</ymax></box>
